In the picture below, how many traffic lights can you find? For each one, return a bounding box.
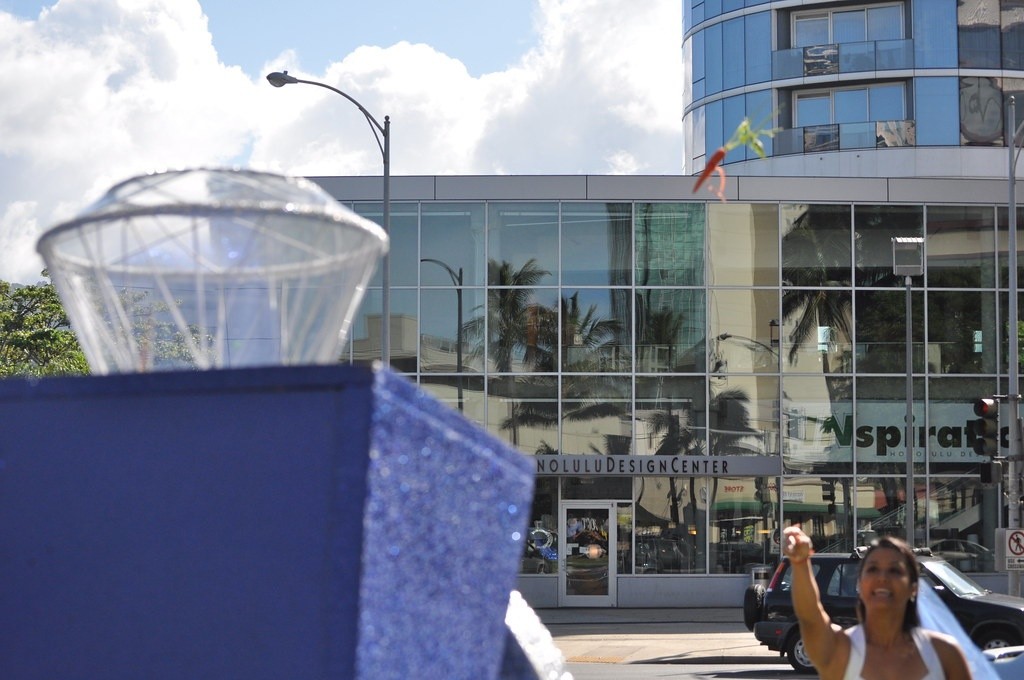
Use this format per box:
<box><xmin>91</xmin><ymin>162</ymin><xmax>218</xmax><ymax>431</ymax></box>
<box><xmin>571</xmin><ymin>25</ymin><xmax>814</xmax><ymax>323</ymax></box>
<box><xmin>981</xmin><ymin>460</ymin><xmax>1003</xmax><ymax>483</ymax></box>
<box><xmin>972</xmin><ymin>398</ymin><xmax>997</xmax><ymax>456</ymax></box>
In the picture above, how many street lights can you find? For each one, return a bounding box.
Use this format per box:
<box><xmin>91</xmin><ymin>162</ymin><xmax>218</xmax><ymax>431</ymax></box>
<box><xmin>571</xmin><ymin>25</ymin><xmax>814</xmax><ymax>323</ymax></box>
<box><xmin>889</xmin><ymin>236</ymin><xmax>926</xmax><ymax>546</ymax></box>
<box><xmin>265</xmin><ymin>68</ymin><xmax>391</xmax><ymax>372</ymax></box>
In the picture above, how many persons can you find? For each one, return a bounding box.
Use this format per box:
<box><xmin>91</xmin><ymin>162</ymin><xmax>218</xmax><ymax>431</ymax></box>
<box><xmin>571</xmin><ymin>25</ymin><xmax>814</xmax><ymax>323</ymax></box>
<box><xmin>780</xmin><ymin>527</ymin><xmax>972</xmax><ymax>680</ymax></box>
<box><xmin>567</xmin><ymin>517</ymin><xmax>585</xmax><ymax>553</ymax></box>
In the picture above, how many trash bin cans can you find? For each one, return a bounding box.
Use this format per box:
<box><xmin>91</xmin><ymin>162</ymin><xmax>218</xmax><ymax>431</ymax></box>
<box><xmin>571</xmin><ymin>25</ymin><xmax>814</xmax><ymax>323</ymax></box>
<box><xmin>751</xmin><ymin>566</ymin><xmax>773</xmax><ymax>592</ymax></box>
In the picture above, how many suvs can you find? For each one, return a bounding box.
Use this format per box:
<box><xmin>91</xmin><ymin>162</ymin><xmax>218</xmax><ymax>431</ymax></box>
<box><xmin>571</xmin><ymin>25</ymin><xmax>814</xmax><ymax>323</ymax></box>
<box><xmin>751</xmin><ymin>546</ymin><xmax>1024</xmax><ymax>674</ymax></box>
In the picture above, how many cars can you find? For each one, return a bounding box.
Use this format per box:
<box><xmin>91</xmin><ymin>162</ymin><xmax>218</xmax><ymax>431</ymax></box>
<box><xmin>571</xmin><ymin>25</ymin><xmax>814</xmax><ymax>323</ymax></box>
<box><xmin>981</xmin><ymin>642</ymin><xmax>1024</xmax><ymax>680</ymax></box>
<box><xmin>523</xmin><ymin>525</ymin><xmax>827</xmax><ymax>588</ymax></box>
<box><xmin>925</xmin><ymin>538</ymin><xmax>994</xmax><ymax>572</ymax></box>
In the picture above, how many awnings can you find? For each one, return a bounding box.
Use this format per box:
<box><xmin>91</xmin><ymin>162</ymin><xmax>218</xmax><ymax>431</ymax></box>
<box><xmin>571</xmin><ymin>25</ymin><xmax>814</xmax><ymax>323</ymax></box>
<box><xmin>712</xmin><ymin>501</ymin><xmax>829</xmax><ymax>515</ymax></box>
<box><xmin>850</xmin><ymin>508</ymin><xmax>881</xmax><ymax>518</ymax></box>
